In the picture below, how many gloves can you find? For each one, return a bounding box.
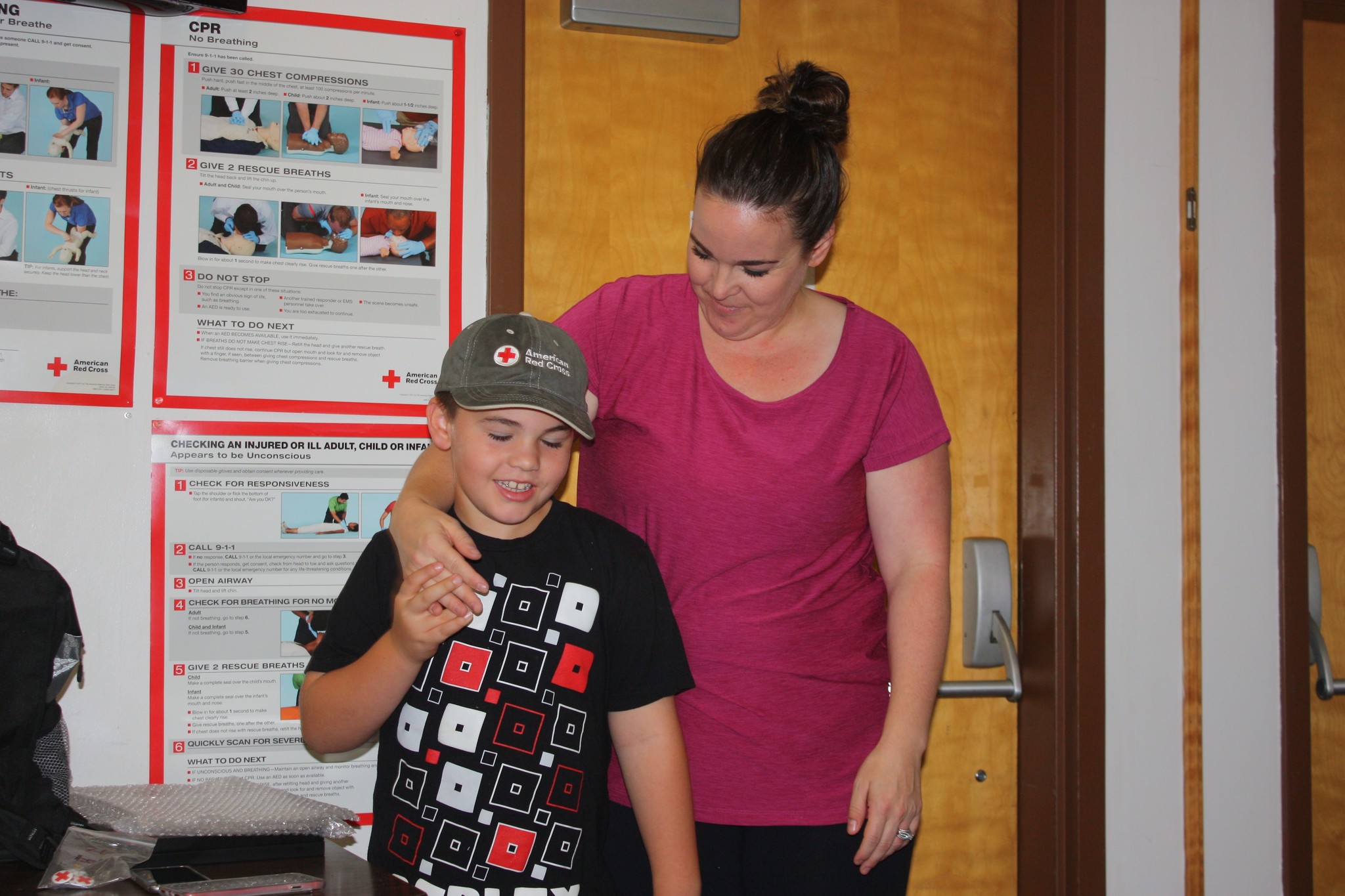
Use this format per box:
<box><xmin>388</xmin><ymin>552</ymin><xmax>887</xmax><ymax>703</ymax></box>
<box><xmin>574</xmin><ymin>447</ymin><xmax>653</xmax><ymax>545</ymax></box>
<box><xmin>225</xmin><ymin>218</ymin><xmax>235</xmax><ymax>234</ymax></box>
<box><xmin>229</xmin><ymin>111</ymin><xmax>247</xmax><ymax>126</ymax></box>
<box><xmin>397</xmin><ymin>239</ymin><xmax>426</xmax><ymax>259</ymax></box>
<box><xmin>385</xmin><ymin>230</ymin><xmax>393</xmax><ymax>238</ymax></box>
<box><xmin>318</xmin><ymin>218</ymin><xmax>332</xmax><ymax>235</ymax></box>
<box><xmin>376</xmin><ymin>109</ymin><xmax>397</xmax><ymax>133</ymax></box>
<box><xmin>243</xmin><ymin>230</ymin><xmax>260</xmax><ymax>245</ymax></box>
<box><xmin>340</xmin><ymin>520</ymin><xmax>347</xmax><ymax>529</ymax></box>
<box><xmin>415</xmin><ymin>121</ymin><xmax>438</xmax><ymax>146</ymax></box>
<box><xmin>302</xmin><ymin>127</ymin><xmax>322</xmax><ymax>146</ymax></box>
<box><xmin>338</xmin><ymin>228</ymin><xmax>353</xmax><ymax>239</ymax></box>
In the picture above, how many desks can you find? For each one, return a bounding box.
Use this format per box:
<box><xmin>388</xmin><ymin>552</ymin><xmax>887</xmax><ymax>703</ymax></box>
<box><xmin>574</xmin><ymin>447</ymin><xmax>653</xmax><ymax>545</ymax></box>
<box><xmin>0</xmin><ymin>838</ymin><xmax>428</xmax><ymax>896</ymax></box>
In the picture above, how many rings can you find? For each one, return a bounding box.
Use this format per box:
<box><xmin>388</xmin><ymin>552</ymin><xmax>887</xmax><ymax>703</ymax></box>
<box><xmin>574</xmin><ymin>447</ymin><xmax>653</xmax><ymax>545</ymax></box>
<box><xmin>896</xmin><ymin>829</ymin><xmax>914</xmax><ymax>841</ymax></box>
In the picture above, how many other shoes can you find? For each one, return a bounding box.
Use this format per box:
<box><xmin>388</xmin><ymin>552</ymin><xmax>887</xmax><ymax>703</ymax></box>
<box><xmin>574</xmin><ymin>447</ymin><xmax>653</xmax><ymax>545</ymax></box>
<box><xmin>281</xmin><ymin>521</ymin><xmax>289</xmax><ymax>534</ymax></box>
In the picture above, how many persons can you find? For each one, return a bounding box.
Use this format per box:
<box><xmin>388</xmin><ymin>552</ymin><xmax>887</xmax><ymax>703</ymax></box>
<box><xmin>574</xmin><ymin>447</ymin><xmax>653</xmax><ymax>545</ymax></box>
<box><xmin>297</xmin><ymin>312</ymin><xmax>709</xmax><ymax>896</ymax></box>
<box><xmin>391</xmin><ymin>58</ymin><xmax>955</xmax><ymax>896</ymax></box>
<box><xmin>285</xmin><ymin>231</ymin><xmax>348</xmax><ymax>254</ymax></box>
<box><xmin>362</xmin><ymin>125</ymin><xmax>427</xmax><ymax>160</ymax></box>
<box><xmin>281</xmin><ymin>202</ymin><xmax>358</xmax><ymax>240</ymax></box>
<box><xmin>361</xmin><ymin>207</ymin><xmax>436</xmax><ymax>259</ymax></box>
<box><xmin>0</xmin><ymin>83</ymin><xmax>28</xmax><ymax>154</ymax></box>
<box><xmin>46</xmin><ymin>87</ymin><xmax>102</xmax><ymax>160</ymax></box>
<box><xmin>286</xmin><ymin>131</ymin><xmax>349</xmax><ymax>156</ymax></box>
<box><xmin>361</xmin><ymin>235</ymin><xmax>408</xmax><ymax>258</ymax></box>
<box><xmin>48</xmin><ymin>226</ymin><xmax>98</xmax><ymax>263</ymax></box>
<box><xmin>44</xmin><ymin>195</ymin><xmax>97</xmax><ymax>266</ymax></box>
<box><xmin>209</xmin><ymin>197</ymin><xmax>278</xmax><ymax>252</ymax></box>
<box><xmin>0</xmin><ymin>190</ymin><xmax>21</xmax><ymax>262</ymax></box>
<box><xmin>201</xmin><ymin>114</ymin><xmax>280</xmax><ymax>155</ymax></box>
<box><xmin>210</xmin><ymin>96</ymin><xmax>262</xmax><ymax>127</ymax></box>
<box><xmin>198</xmin><ymin>227</ymin><xmax>256</xmax><ymax>256</ymax></box>
<box><xmin>287</xmin><ymin>102</ymin><xmax>333</xmax><ymax>146</ymax></box>
<box><xmin>48</xmin><ymin>125</ymin><xmax>86</xmax><ymax>159</ymax></box>
<box><xmin>376</xmin><ymin>109</ymin><xmax>438</xmax><ymax>147</ymax></box>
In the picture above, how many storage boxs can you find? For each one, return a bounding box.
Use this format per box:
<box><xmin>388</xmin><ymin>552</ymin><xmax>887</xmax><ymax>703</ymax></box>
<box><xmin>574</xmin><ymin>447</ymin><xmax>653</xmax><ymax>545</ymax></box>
<box><xmin>91</xmin><ymin>823</ymin><xmax>325</xmax><ymax>869</ymax></box>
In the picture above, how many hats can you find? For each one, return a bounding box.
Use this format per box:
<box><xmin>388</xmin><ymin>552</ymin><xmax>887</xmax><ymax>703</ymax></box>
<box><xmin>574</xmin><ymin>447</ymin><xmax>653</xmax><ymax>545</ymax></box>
<box><xmin>433</xmin><ymin>311</ymin><xmax>595</xmax><ymax>442</ymax></box>
